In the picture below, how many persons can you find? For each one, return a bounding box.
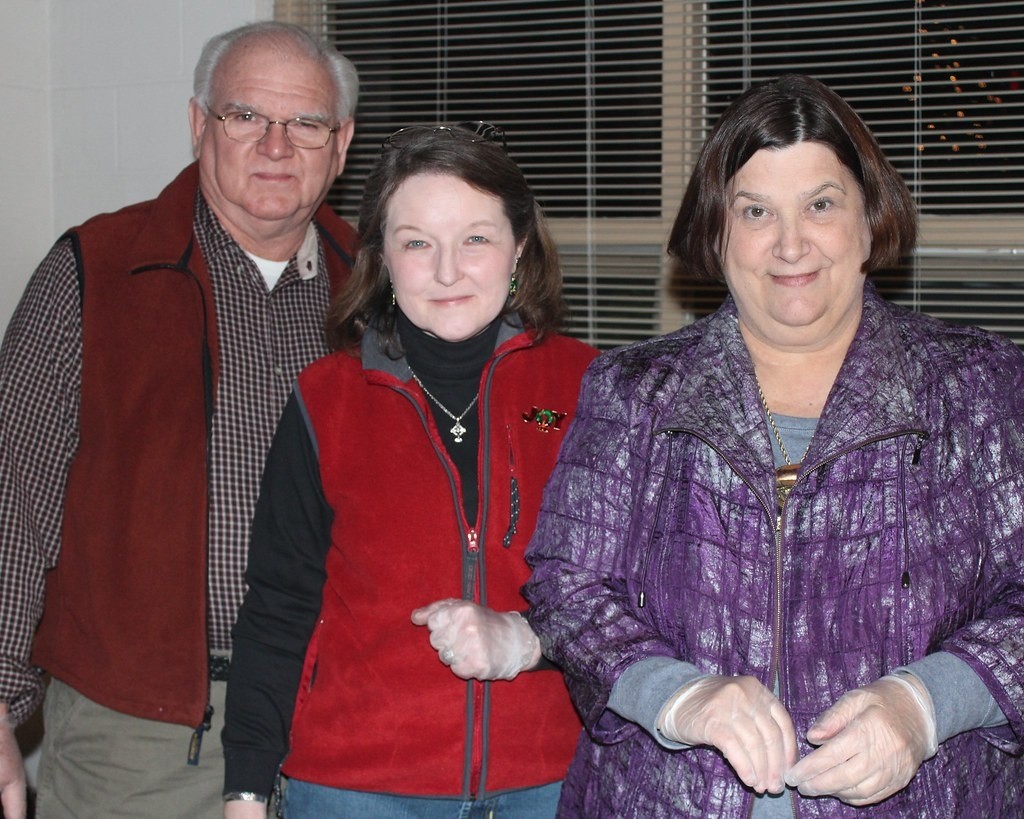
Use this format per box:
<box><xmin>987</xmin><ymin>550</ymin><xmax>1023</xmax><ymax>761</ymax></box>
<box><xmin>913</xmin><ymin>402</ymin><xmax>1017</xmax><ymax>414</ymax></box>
<box><xmin>2</xmin><ymin>18</ymin><xmax>368</xmax><ymax>819</ymax></box>
<box><xmin>519</xmin><ymin>68</ymin><xmax>1024</xmax><ymax>817</ymax></box>
<box><xmin>217</xmin><ymin>121</ymin><xmax>582</xmax><ymax>819</ymax></box>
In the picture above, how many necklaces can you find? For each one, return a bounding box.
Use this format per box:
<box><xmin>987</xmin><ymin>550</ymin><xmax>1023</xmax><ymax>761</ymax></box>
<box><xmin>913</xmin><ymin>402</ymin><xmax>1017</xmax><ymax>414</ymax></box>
<box><xmin>408</xmin><ymin>359</ymin><xmax>478</xmax><ymax>443</ymax></box>
<box><xmin>753</xmin><ymin>370</ymin><xmax>825</xmax><ymax>511</ymax></box>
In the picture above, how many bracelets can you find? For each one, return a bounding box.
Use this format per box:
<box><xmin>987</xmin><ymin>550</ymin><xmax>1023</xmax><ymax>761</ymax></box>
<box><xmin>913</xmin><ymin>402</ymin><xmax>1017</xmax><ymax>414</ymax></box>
<box><xmin>223</xmin><ymin>791</ymin><xmax>269</xmax><ymax>803</ymax></box>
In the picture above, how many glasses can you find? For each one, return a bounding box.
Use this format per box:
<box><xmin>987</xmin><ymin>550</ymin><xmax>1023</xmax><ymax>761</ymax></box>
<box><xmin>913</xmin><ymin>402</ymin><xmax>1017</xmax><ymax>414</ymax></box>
<box><xmin>382</xmin><ymin>121</ymin><xmax>507</xmax><ymax>150</ymax></box>
<box><xmin>205</xmin><ymin>101</ymin><xmax>340</xmax><ymax>149</ymax></box>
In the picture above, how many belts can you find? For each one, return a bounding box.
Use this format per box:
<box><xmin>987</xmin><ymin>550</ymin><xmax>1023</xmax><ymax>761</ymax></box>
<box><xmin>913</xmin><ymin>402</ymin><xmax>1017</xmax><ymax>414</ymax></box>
<box><xmin>209</xmin><ymin>654</ymin><xmax>230</xmax><ymax>682</ymax></box>
